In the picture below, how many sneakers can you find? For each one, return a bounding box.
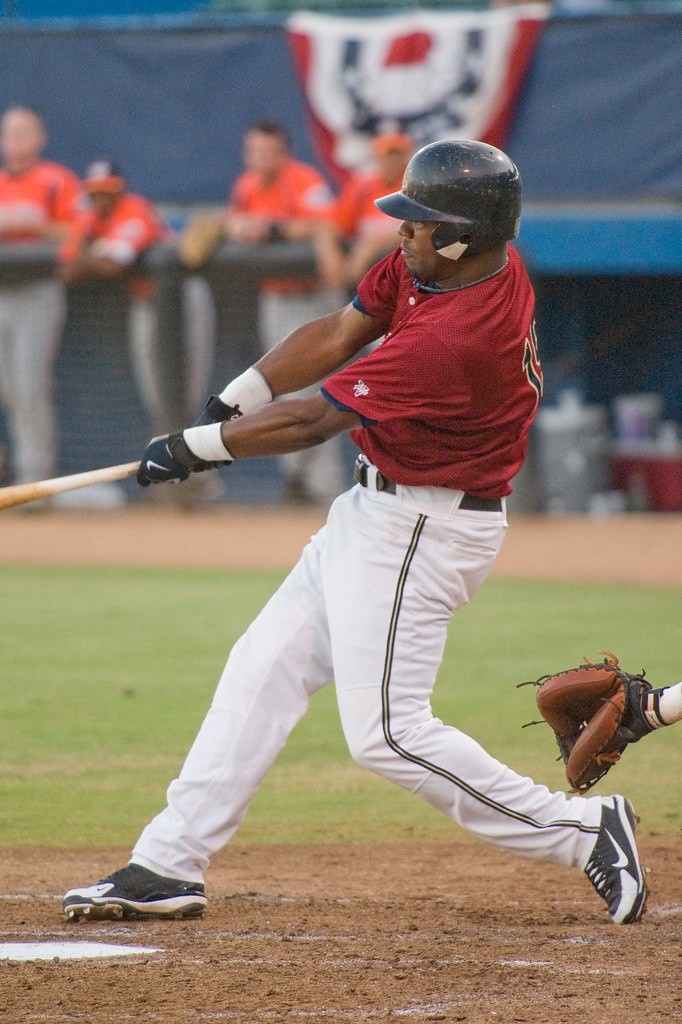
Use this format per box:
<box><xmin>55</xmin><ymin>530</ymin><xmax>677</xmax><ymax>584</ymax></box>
<box><xmin>584</xmin><ymin>794</ymin><xmax>650</xmax><ymax>924</ymax></box>
<box><xmin>62</xmin><ymin>864</ymin><xmax>208</xmax><ymax>921</ymax></box>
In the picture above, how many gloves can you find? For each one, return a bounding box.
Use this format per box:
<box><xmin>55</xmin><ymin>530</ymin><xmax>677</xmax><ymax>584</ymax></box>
<box><xmin>137</xmin><ymin>422</ymin><xmax>235</xmax><ymax>486</ymax></box>
<box><xmin>192</xmin><ymin>366</ymin><xmax>275</xmax><ymax>472</ymax></box>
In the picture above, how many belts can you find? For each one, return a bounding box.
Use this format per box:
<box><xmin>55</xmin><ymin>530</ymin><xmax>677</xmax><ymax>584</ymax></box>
<box><xmin>354</xmin><ymin>459</ymin><xmax>502</xmax><ymax>512</ymax></box>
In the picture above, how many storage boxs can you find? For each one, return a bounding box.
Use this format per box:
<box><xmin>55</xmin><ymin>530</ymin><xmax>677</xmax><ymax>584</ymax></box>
<box><xmin>610</xmin><ymin>448</ymin><xmax>682</xmax><ymax>514</ymax></box>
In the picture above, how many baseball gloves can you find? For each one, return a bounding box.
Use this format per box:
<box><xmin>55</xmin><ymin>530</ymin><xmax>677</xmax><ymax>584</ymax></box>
<box><xmin>515</xmin><ymin>651</ymin><xmax>672</xmax><ymax>796</ymax></box>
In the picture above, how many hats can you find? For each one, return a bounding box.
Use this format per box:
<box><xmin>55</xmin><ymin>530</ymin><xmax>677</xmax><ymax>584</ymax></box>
<box><xmin>84</xmin><ymin>163</ymin><xmax>123</xmax><ymax>192</ymax></box>
<box><xmin>373</xmin><ymin>134</ymin><xmax>411</xmax><ymax>153</ymax></box>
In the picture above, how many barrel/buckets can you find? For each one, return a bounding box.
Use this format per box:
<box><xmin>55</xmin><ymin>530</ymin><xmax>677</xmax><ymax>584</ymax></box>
<box><xmin>612</xmin><ymin>393</ymin><xmax>664</xmax><ymax>448</ymax></box>
<box><xmin>534</xmin><ymin>392</ymin><xmax>613</xmax><ymax>512</ymax></box>
<box><xmin>616</xmin><ymin>449</ymin><xmax>682</xmax><ymax>511</ymax></box>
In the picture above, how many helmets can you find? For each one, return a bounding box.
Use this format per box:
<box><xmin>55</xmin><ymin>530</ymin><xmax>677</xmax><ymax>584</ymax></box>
<box><xmin>374</xmin><ymin>139</ymin><xmax>522</xmax><ymax>260</ymax></box>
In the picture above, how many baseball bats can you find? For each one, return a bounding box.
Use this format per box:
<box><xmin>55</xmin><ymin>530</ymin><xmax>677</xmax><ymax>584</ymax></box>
<box><xmin>0</xmin><ymin>458</ymin><xmax>143</xmax><ymax>510</ymax></box>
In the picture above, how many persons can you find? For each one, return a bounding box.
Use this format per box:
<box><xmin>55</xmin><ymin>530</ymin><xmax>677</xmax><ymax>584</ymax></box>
<box><xmin>1</xmin><ymin>107</ymin><xmax>414</xmax><ymax>511</ymax></box>
<box><xmin>63</xmin><ymin>141</ymin><xmax>651</xmax><ymax>931</ymax></box>
<box><xmin>613</xmin><ymin>681</ymin><xmax>682</xmax><ymax>744</ymax></box>
